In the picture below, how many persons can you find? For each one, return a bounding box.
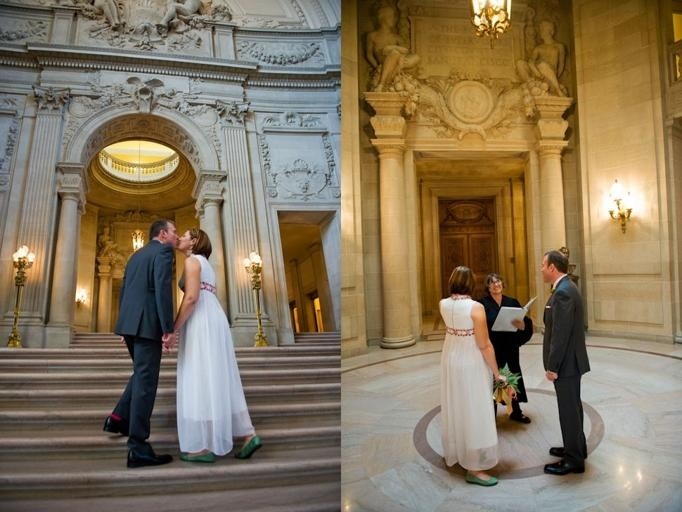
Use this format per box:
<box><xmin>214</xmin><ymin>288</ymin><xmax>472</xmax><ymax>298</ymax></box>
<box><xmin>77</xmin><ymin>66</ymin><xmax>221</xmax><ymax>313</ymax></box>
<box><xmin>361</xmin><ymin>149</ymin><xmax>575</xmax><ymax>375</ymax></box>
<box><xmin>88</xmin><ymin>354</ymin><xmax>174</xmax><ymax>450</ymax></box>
<box><xmin>543</xmin><ymin>251</ymin><xmax>590</xmax><ymax>474</ymax></box>
<box><xmin>156</xmin><ymin>0</ymin><xmax>200</xmax><ymax>29</ymax></box>
<box><xmin>515</xmin><ymin>20</ymin><xmax>566</xmax><ymax>97</ymax></box>
<box><xmin>93</xmin><ymin>0</ymin><xmax>120</xmax><ymax>29</ymax></box>
<box><xmin>366</xmin><ymin>7</ymin><xmax>421</xmax><ymax>91</ymax></box>
<box><xmin>478</xmin><ymin>273</ymin><xmax>534</xmax><ymax>423</ymax></box>
<box><xmin>439</xmin><ymin>264</ymin><xmax>506</xmax><ymax>487</ymax></box>
<box><xmin>162</xmin><ymin>227</ymin><xmax>263</xmax><ymax>464</ymax></box>
<box><xmin>103</xmin><ymin>219</ymin><xmax>177</xmax><ymax>467</ymax></box>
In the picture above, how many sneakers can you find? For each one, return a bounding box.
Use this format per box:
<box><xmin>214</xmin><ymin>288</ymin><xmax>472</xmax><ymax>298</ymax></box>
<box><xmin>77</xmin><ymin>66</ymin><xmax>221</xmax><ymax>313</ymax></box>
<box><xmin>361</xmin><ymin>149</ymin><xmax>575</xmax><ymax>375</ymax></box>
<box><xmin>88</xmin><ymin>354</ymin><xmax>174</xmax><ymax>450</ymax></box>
<box><xmin>510</xmin><ymin>413</ymin><xmax>531</xmax><ymax>426</ymax></box>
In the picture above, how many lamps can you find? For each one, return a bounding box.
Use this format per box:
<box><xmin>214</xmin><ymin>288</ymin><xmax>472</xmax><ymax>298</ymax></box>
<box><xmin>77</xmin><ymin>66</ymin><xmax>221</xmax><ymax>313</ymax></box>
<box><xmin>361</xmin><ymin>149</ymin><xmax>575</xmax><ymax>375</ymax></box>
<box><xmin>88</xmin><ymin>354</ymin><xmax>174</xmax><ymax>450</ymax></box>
<box><xmin>131</xmin><ymin>143</ymin><xmax>145</xmax><ymax>251</ymax></box>
<box><xmin>470</xmin><ymin>0</ymin><xmax>512</xmax><ymax>48</ymax></box>
<box><xmin>75</xmin><ymin>287</ymin><xmax>87</xmax><ymax>308</ymax></box>
<box><xmin>607</xmin><ymin>177</ymin><xmax>632</xmax><ymax>234</ymax></box>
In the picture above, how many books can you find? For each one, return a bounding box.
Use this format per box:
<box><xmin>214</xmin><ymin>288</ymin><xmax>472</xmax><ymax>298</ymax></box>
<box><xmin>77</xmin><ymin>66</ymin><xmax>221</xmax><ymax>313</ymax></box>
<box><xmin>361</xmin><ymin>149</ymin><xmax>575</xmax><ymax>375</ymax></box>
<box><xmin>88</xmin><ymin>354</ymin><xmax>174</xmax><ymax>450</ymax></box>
<box><xmin>491</xmin><ymin>296</ymin><xmax>538</xmax><ymax>333</ymax></box>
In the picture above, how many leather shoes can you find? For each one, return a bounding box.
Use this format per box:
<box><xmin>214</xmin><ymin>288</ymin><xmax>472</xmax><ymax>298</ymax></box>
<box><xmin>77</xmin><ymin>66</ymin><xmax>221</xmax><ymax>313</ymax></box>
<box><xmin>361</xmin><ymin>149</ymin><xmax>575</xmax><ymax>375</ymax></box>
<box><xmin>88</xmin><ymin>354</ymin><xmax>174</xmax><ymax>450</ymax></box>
<box><xmin>234</xmin><ymin>436</ymin><xmax>263</xmax><ymax>461</ymax></box>
<box><xmin>549</xmin><ymin>445</ymin><xmax>587</xmax><ymax>458</ymax></box>
<box><xmin>464</xmin><ymin>474</ymin><xmax>498</xmax><ymax>488</ymax></box>
<box><xmin>126</xmin><ymin>445</ymin><xmax>174</xmax><ymax>468</ymax></box>
<box><xmin>544</xmin><ymin>457</ymin><xmax>585</xmax><ymax>476</ymax></box>
<box><xmin>179</xmin><ymin>452</ymin><xmax>216</xmax><ymax>464</ymax></box>
<box><xmin>102</xmin><ymin>415</ymin><xmax>128</xmax><ymax>436</ymax></box>
